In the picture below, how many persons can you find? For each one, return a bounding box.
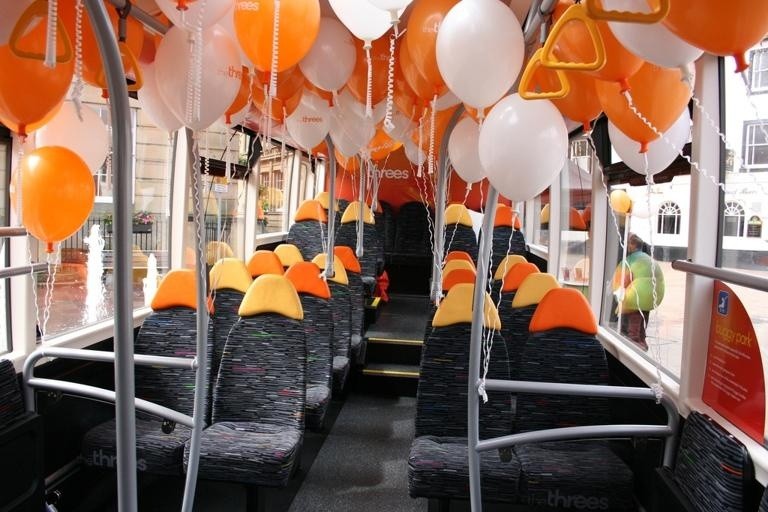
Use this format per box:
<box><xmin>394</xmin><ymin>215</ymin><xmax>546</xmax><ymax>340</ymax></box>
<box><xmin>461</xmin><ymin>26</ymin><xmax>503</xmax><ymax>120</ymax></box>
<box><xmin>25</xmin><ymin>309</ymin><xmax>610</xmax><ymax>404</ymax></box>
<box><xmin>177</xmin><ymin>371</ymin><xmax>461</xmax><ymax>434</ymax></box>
<box><xmin>608</xmin><ymin>231</ymin><xmax>654</xmax><ymax>352</ymax></box>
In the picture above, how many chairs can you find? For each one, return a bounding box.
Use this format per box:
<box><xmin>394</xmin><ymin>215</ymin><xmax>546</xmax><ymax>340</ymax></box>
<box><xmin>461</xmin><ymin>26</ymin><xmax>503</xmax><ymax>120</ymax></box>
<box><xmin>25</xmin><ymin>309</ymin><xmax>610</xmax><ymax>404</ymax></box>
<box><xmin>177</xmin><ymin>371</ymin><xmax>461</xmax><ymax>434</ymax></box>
<box><xmin>181</xmin><ymin>275</ymin><xmax>305</xmax><ymax>512</ymax></box>
<box><xmin>200</xmin><ymin>190</ymin><xmax>438</xmax><ymax>436</ymax></box>
<box><xmin>77</xmin><ymin>269</ymin><xmax>215</xmax><ymax>510</ymax></box>
<box><xmin>408</xmin><ymin>191</ymin><xmax>768</xmax><ymax>512</ymax></box>
